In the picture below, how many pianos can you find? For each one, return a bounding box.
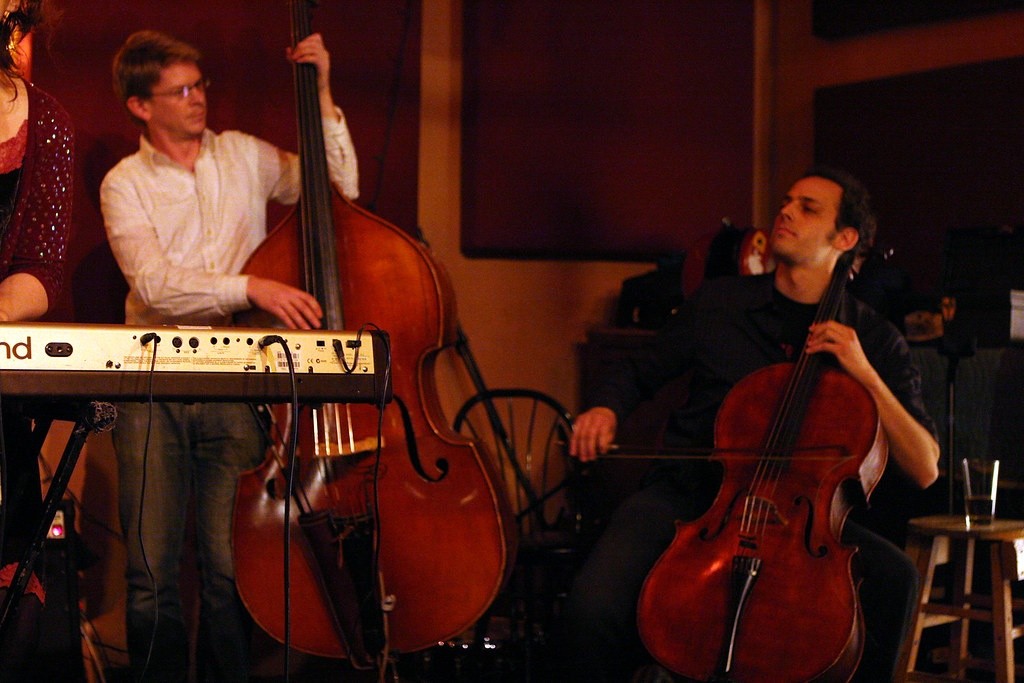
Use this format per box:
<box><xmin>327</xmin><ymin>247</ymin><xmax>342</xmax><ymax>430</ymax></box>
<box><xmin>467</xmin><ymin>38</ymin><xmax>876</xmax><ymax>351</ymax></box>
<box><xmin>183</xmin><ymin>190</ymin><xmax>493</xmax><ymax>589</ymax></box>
<box><xmin>2</xmin><ymin>321</ymin><xmax>395</xmax><ymax>683</ymax></box>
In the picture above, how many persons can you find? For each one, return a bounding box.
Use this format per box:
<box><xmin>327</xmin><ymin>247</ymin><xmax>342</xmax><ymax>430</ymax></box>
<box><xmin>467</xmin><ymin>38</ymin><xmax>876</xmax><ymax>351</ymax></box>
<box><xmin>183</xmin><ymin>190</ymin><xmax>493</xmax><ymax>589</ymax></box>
<box><xmin>0</xmin><ymin>0</ymin><xmax>76</xmax><ymax>559</ymax></box>
<box><xmin>101</xmin><ymin>30</ymin><xmax>359</xmax><ymax>683</ymax></box>
<box><xmin>554</xmin><ymin>168</ymin><xmax>940</xmax><ymax>683</ymax></box>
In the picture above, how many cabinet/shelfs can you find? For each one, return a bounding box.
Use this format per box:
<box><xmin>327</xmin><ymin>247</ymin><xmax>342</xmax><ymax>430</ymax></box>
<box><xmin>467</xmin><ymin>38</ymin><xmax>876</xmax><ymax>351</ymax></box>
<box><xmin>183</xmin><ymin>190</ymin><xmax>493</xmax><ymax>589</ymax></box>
<box><xmin>575</xmin><ymin>325</ymin><xmax>678</xmax><ymax>487</ymax></box>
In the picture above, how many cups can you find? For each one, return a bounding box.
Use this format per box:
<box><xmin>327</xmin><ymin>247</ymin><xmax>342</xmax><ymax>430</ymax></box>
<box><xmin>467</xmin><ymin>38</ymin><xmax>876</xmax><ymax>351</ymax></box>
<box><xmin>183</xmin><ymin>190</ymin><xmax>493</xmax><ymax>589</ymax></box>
<box><xmin>962</xmin><ymin>456</ymin><xmax>999</xmax><ymax>529</ymax></box>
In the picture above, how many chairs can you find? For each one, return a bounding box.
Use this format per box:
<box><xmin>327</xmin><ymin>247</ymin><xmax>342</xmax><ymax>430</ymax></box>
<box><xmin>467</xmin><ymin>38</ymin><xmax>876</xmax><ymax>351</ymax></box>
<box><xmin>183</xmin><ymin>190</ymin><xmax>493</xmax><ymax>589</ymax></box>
<box><xmin>451</xmin><ymin>388</ymin><xmax>593</xmax><ymax>682</ymax></box>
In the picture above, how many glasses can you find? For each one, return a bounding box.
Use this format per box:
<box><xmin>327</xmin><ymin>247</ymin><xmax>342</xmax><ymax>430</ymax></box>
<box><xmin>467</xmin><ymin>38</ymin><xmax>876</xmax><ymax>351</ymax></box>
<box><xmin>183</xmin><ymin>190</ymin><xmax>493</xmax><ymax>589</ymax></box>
<box><xmin>148</xmin><ymin>76</ymin><xmax>211</xmax><ymax>98</ymax></box>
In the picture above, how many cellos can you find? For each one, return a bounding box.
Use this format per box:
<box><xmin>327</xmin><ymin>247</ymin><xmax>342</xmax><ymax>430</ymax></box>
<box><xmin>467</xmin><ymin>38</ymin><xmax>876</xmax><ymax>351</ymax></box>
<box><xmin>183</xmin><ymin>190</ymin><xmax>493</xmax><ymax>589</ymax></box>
<box><xmin>632</xmin><ymin>226</ymin><xmax>898</xmax><ymax>679</ymax></box>
<box><xmin>228</xmin><ymin>0</ymin><xmax>524</xmax><ymax>671</ymax></box>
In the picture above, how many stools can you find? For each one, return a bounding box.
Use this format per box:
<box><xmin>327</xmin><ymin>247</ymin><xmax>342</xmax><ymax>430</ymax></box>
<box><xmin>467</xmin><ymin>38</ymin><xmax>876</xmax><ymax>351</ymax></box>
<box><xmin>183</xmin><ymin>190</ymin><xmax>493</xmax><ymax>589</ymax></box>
<box><xmin>889</xmin><ymin>510</ymin><xmax>1024</xmax><ymax>683</ymax></box>
